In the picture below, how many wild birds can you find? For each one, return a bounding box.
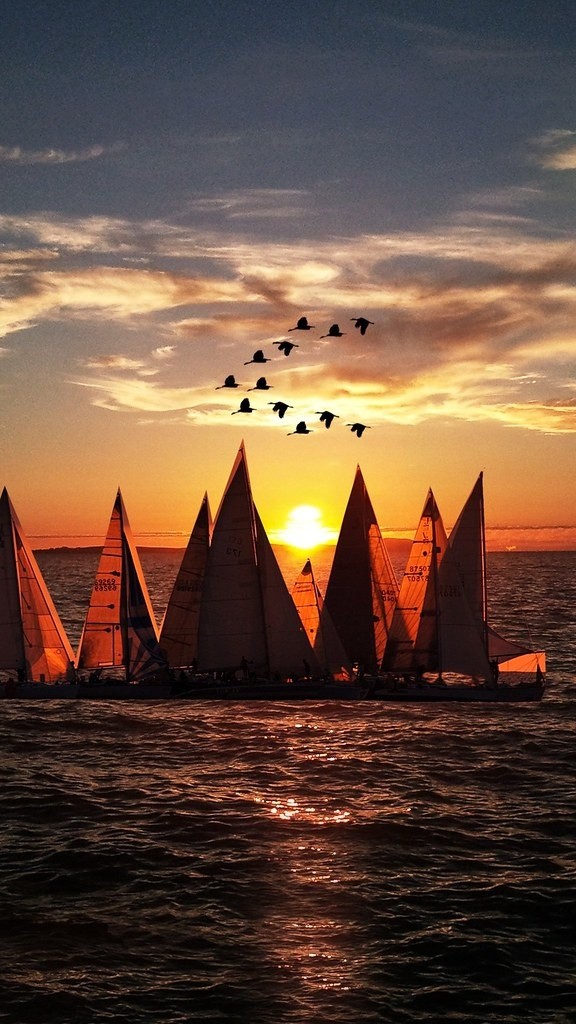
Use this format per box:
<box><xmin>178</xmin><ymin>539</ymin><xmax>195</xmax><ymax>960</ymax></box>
<box><xmin>247</xmin><ymin>377</ymin><xmax>273</xmax><ymax>392</ymax></box>
<box><xmin>230</xmin><ymin>398</ymin><xmax>257</xmax><ymax>415</ymax></box>
<box><xmin>268</xmin><ymin>402</ymin><xmax>294</xmax><ymax>418</ymax></box>
<box><xmin>286</xmin><ymin>421</ymin><xmax>314</xmax><ymax>436</ymax></box>
<box><xmin>350</xmin><ymin>317</ymin><xmax>374</xmax><ymax>335</ymax></box>
<box><xmin>320</xmin><ymin>323</ymin><xmax>347</xmax><ymax>339</ymax></box>
<box><xmin>214</xmin><ymin>375</ymin><xmax>240</xmax><ymax>390</ymax></box>
<box><xmin>314</xmin><ymin>411</ymin><xmax>340</xmax><ymax>429</ymax></box>
<box><xmin>287</xmin><ymin>317</ymin><xmax>315</xmax><ymax>332</ymax></box>
<box><xmin>272</xmin><ymin>341</ymin><xmax>300</xmax><ymax>356</ymax></box>
<box><xmin>243</xmin><ymin>350</ymin><xmax>271</xmax><ymax>365</ymax></box>
<box><xmin>345</xmin><ymin>423</ymin><xmax>371</xmax><ymax>438</ymax></box>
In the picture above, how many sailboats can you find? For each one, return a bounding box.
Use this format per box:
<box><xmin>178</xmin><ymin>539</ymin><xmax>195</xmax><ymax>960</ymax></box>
<box><xmin>1</xmin><ymin>440</ymin><xmax>547</xmax><ymax>702</ymax></box>
